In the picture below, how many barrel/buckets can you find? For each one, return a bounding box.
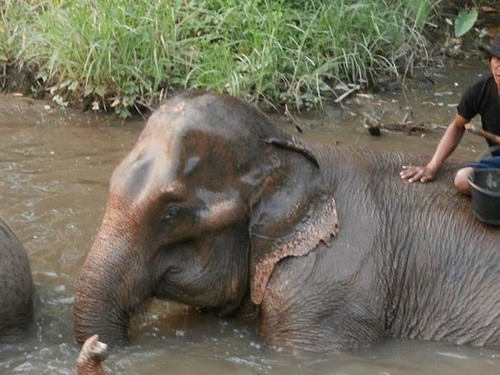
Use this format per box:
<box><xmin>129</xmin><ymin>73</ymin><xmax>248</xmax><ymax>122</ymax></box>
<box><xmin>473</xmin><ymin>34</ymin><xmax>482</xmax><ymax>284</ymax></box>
<box><xmin>466</xmin><ymin>144</ymin><xmax>499</xmax><ymax>227</ymax></box>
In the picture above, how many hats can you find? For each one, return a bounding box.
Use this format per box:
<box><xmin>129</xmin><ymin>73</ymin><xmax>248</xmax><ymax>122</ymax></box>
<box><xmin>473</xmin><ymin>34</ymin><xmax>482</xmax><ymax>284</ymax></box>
<box><xmin>477</xmin><ymin>34</ymin><xmax>500</xmax><ymax>60</ymax></box>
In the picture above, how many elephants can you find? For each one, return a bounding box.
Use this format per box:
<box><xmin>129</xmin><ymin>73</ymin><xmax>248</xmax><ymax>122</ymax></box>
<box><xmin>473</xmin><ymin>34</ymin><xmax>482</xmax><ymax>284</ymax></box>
<box><xmin>73</xmin><ymin>87</ymin><xmax>500</xmax><ymax>375</ymax></box>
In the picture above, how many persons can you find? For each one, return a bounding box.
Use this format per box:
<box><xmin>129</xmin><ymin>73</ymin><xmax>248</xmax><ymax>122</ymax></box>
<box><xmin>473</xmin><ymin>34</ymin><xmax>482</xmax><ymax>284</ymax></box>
<box><xmin>399</xmin><ymin>31</ymin><xmax>500</xmax><ymax>197</ymax></box>
